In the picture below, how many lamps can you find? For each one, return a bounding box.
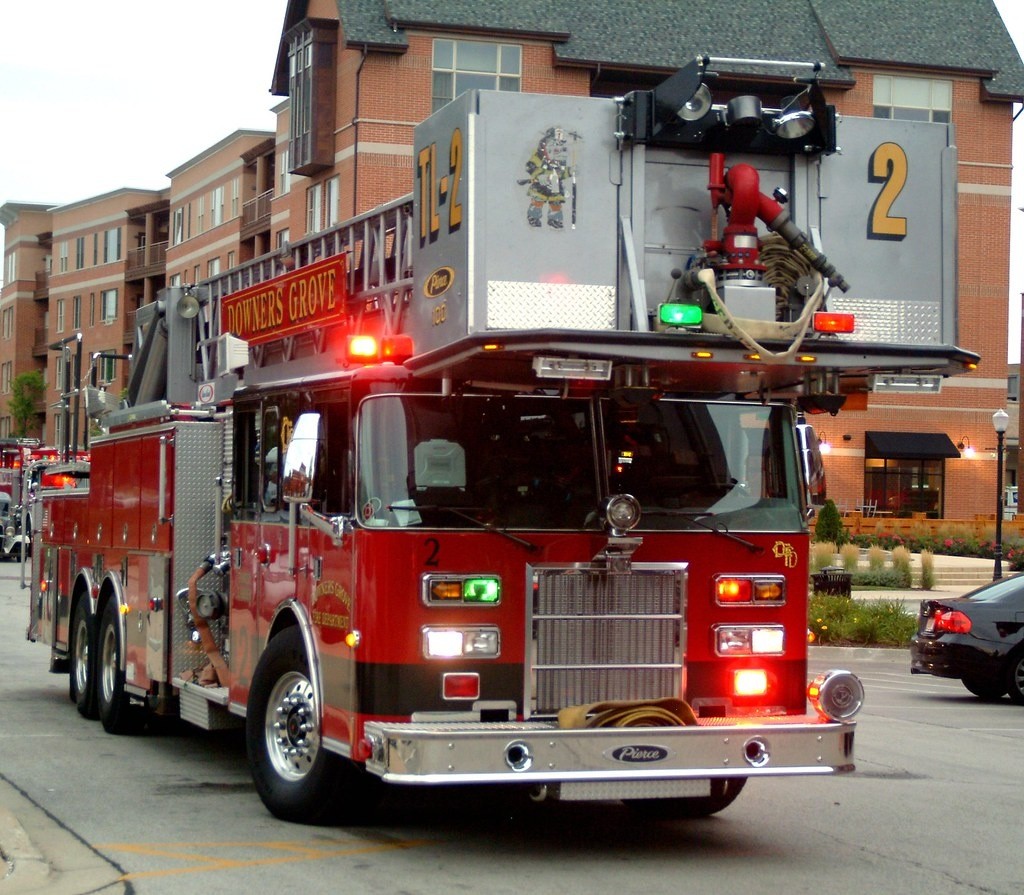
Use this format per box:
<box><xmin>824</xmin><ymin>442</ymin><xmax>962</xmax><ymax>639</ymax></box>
<box><xmin>818</xmin><ymin>431</ymin><xmax>831</xmax><ymax>454</ymax></box>
<box><xmin>958</xmin><ymin>435</ymin><xmax>975</xmax><ymax>458</ymax></box>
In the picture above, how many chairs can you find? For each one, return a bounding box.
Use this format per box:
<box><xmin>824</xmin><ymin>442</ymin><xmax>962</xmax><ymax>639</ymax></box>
<box><xmin>832</xmin><ymin>496</ymin><xmax>882</xmax><ymax>518</ymax></box>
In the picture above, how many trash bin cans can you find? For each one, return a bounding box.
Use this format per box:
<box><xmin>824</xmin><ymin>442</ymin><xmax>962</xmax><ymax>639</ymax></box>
<box><xmin>810</xmin><ymin>566</ymin><xmax>853</xmax><ymax>601</ymax></box>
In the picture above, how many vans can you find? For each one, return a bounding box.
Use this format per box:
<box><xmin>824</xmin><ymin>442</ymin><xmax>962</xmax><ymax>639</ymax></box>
<box><xmin>1003</xmin><ymin>485</ymin><xmax>1019</xmax><ymax>522</ymax></box>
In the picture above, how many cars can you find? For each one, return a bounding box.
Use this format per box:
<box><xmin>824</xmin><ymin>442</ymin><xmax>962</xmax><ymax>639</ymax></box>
<box><xmin>908</xmin><ymin>572</ymin><xmax>1024</xmax><ymax>707</ymax></box>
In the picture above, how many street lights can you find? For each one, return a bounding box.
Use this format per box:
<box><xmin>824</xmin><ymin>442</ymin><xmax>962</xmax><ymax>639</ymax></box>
<box><xmin>991</xmin><ymin>406</ymin><xmax>1011</xmax><ymax>584</ymax></box>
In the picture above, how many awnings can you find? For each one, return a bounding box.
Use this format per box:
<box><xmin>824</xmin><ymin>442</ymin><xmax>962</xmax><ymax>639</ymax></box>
<box><xmin>866</xmin><ymin>431</ymin><xmax>961</xmax><ymax>458</ymax></box>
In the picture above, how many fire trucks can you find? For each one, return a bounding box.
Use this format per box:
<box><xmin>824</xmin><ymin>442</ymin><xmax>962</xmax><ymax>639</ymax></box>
<box><xmin>1</xmin><ymin>436</ymin><xmax>95</xmax><ymax>564</ymax></box>
<box><xmin>17</xmin><ymin>53</ymin><xmax>983</xmax><ymax>829</ymax></box>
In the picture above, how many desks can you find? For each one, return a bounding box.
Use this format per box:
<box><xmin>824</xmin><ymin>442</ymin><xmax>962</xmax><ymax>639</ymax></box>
<box><xmin>872</xmin><ymin>511</ymin><xmax>895</xmax><ymax>517</ymax></box>
